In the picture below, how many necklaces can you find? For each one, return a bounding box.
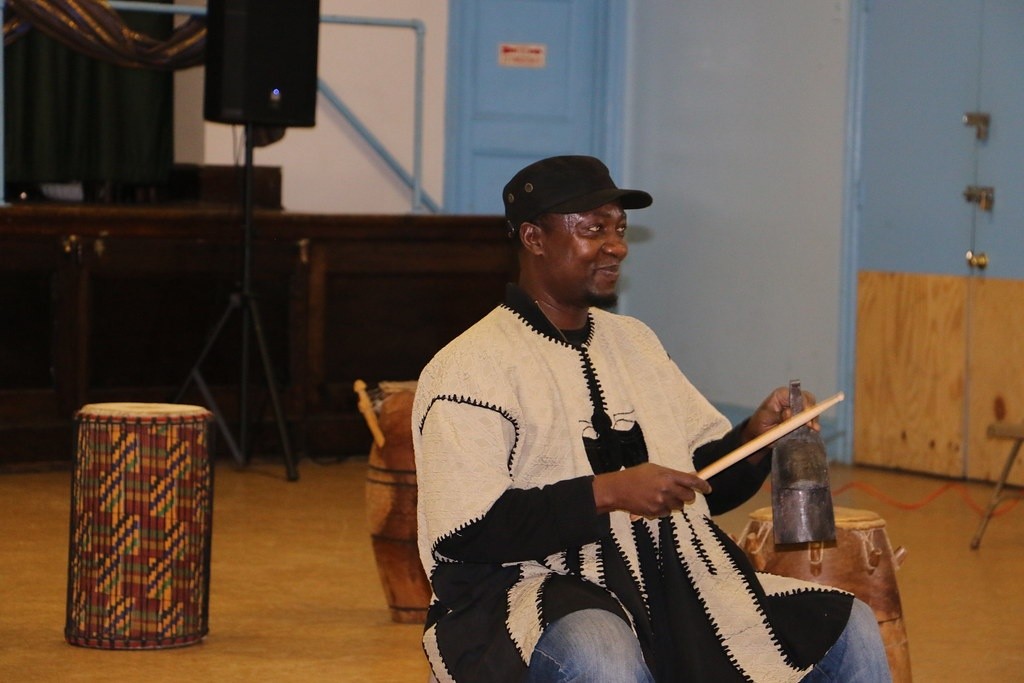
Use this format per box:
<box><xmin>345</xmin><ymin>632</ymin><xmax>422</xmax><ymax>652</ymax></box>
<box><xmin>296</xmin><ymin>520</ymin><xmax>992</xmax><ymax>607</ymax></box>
<box><xmin>535</xmin><ymin>300</ymin><xmax>568</xmax><ymax>342</ymax></box>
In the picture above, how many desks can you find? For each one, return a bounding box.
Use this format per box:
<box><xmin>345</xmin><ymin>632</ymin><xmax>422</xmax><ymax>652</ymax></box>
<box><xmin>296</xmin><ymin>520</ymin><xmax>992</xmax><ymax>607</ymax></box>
<box><xmin>0</xmin><ymin>203</ymin><xmax>520</xmax><ymax>464</ymax></box>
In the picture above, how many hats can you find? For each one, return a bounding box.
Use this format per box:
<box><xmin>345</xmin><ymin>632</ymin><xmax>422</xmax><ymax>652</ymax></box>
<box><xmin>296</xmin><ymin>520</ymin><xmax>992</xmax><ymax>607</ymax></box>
<box><xmin>500</xmin><ymin>155</ymin><xmax>655</xmax><ymax>239</ymax></box>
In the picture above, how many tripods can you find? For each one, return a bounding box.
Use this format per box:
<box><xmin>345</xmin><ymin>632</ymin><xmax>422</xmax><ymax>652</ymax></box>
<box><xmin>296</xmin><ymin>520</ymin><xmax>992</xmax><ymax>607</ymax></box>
<box><xmin>178</xmin><ymin>123</ymin><xmax>300</xmax><ymax>484</ymax></box>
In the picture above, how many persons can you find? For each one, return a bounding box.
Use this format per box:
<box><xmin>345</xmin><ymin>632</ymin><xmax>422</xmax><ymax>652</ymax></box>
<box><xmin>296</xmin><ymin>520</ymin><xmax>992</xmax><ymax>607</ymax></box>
<box><xmin>411</xmin><ymin>155</ymin><xmax>892</xmax><ymax>683</ymax></box>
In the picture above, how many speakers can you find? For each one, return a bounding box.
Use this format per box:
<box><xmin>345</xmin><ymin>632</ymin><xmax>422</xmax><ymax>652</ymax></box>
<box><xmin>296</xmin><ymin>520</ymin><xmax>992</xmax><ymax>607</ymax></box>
<box><xmin>204</xmin><ymin>1</ymin><xmax>320</xmax><ymax>128</ymax></box>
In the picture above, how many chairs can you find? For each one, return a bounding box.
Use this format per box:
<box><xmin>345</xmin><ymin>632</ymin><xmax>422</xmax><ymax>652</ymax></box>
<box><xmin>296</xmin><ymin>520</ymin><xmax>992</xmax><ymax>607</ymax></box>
<box><xmin>970</xmin><ymin>422</ymin><xmax>1024</xmax><ymax>548</ymax></box>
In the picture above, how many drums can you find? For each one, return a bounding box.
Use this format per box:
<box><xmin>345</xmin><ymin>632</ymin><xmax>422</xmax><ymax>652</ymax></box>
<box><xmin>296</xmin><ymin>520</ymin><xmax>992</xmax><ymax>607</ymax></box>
<box><xmin>62</xmin><ymin>402</ymin><xmax>218</xmax><ymax>652</ymax></box>
<box><xmin>748</xmin><ymin>501</ymin><xmax>914</xmax><ymax>683</ymax></box>
<box><xmin>363</xmin><ymin>379</ymin><xmax>435</xmax><ymax>627</ymax></box>
